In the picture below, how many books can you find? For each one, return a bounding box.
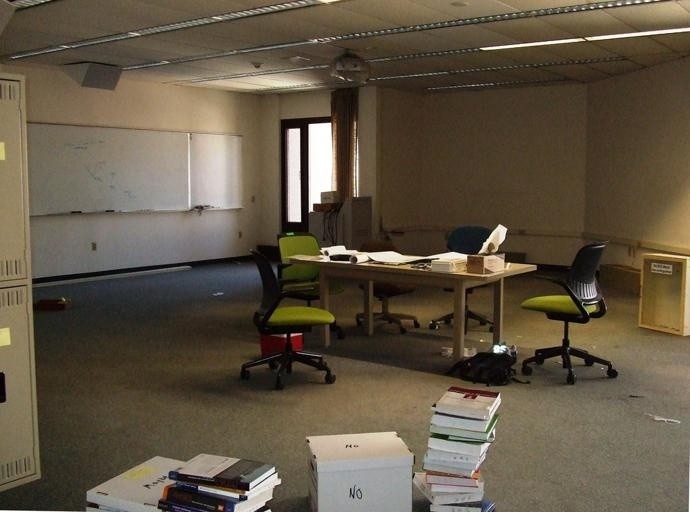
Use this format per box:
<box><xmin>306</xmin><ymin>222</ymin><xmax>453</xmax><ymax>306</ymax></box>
<box><xmin>158</xmin><ymin>452</ymin><xmax>283</xmax><ymax>511</ymax></box>
<box><xmin>414</xmin><ymin>384</ymin><xmax>502</xmax><ymax>512</ymax></box>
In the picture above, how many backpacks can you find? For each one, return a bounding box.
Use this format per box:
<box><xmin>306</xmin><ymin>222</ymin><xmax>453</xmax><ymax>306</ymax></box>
<box><xmin>445</xmin><ymin>352</ymin><xmax>516</xmax><ymax>387</ymax></box>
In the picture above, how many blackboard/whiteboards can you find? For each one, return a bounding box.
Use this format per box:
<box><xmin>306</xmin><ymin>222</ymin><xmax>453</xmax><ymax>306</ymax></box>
<box><xmin>26</xmin><ymin>120</ymin><xmax>245</xmax><ymax>217</ymax></box>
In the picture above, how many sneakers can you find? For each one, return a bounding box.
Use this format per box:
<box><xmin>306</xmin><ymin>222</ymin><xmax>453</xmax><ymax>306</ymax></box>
<box><xmin>487</xmin><ymin>341</ymin><xmax>518</xmax><ymax>358</ymax></box>
<box><xmin>441</xmin><ymin>344</ymin><xmax>476</xmax><ymax>357</ymax></box>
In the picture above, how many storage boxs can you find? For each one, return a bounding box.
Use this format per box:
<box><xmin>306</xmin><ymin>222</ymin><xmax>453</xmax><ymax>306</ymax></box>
<box><xmin>304</xmin><ymin>432</ymin><xmax>416</xmax><ymax>512</ymax></box>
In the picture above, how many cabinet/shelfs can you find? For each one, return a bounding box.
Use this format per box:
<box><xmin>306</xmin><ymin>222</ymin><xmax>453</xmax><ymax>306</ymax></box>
<box><xmin>0</xmin><ymin>73</ymin><xmax>43</xmax><ymax>493</ymax></box>
<box><xmin>637</xmin><ymin>254</ymin><xmax>690</xmax><ymax>337</ymax></box>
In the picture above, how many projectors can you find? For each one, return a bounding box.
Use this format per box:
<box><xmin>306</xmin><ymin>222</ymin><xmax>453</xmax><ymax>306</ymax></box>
<box><xmin>329</xmin><ymin>55</ymin><xmax>368</xmax><ymax>72</ymax></box>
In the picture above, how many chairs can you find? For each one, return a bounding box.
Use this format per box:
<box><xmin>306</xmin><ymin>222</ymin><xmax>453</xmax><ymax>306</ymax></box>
<box><xmin>240</xmin><ymin>249</ymin><xmax>335</xmax><ymax>390</ymax></box>
<box><xmin>276</xmin><ymin>232</ymin><xmax>345</xmax><ymax>343</ymax></box>
<box><xmin>430</xmin><ymin>226</ymin><xmax>495</xmax><ymax>334</ymax></box>
<box><xmin>356</xmin><ymin>241</ymin><xmax>421</xmax><ymax>334</ymax></box>
<box><xmin>521</xmin><ymin>243</ymin><xmax>618</xmax><ymax>384</ymax></box>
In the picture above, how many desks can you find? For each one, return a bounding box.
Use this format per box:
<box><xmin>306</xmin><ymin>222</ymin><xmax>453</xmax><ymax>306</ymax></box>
<box><xmin>287</xmin><ymin>250</ymin><xmax>538</xmax><ymax>362</ymax></box>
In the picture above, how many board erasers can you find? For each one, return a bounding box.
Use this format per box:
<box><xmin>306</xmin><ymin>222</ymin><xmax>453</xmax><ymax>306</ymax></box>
<box><xmin>105</xmin><ymin>210</ymin><xmax>115</xmax><ymax>212</ymax></box>
<box><xmin>71</xmin><ymin>211</ymin><xmax>82</xmax><ymax>213</ymax></box>
<box><xmin>195</xmin><ymin>205</ymin><xmax>209</xmax><ymax>209</ymax></box>
